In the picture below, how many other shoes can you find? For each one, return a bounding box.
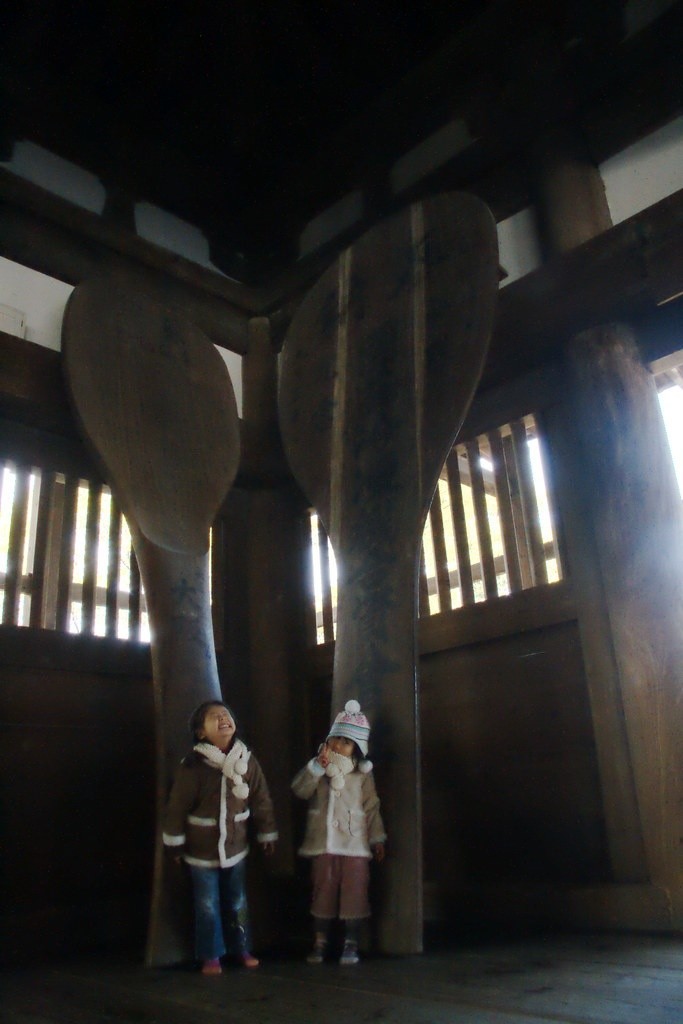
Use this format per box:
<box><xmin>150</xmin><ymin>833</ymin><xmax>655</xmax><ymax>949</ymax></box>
<box><xmin>306</xmin><ymin>940</ymin><xmax>329</xmax><ymax>962</ymax></box>
<box><xmin>238</xmin><ymin>952</ymin><xmax>260</xmax><ymax>967</ymax></box>
<box><xmin>201</xmin><ymin>959</ymin><xmax>222</xmax><ymax>976</ymax></box>
<box><xmin>338</xmin><ymin>939</ymin><xmax>359</xmax><ymax>966</ymax></box>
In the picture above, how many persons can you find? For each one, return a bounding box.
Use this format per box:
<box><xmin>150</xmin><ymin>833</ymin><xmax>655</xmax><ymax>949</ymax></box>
<box><xmin>163</xmin><ymin>701</ymin><xmax>278</xmax><ymax>976</ymax></box>
<box><xmin>292</xmin><ymin>712</ymin><xmax>387</xmax><ymax>964</ymax></box>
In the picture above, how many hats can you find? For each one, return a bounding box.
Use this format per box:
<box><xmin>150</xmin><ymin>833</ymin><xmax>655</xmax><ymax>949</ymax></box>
<box><xmin>317</xmin><ymin>700</ymin><xmax>373</xmax><ymax>774</ymax></box>
